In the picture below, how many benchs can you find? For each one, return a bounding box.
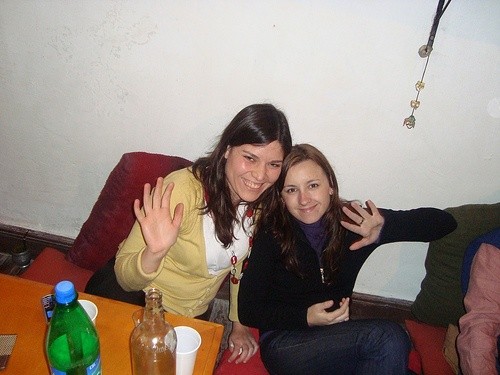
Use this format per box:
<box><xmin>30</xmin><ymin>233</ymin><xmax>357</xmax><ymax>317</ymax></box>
<box><xmin>18</xmin><ymin>245</ymin><xmax>421</xmax><ymax>375</ymax></box>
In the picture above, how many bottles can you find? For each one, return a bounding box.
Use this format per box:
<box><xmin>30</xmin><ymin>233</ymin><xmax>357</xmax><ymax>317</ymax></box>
<box><xmin>130</xmin><ymin>288</ymin><xmax>177</xmax><ymax>375</ymax></box>
<box><xmin>46</xmin><ymin>281</ymin><xmax>102</xmax><ymax>375</ymax></box>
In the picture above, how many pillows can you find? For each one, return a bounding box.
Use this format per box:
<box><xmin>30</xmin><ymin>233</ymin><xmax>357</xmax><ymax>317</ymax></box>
<box><xmin>65</xmin><ymin>151</ymin><xmax>195</xmax><ymax>272</ymax></box>
<box><xmin>405</xmin><ymin>321</ymin><xmax>458</xmax><ymax>375</ymax></box>
<box><xmin>413</xmin><ymin>201</ymin><xmax>500</xmax><ymax>326</ymax></box>
<box><xmin>457</xmin><ymin>243</ymin><xmax>500</xmax><ymax>375</ymax></box>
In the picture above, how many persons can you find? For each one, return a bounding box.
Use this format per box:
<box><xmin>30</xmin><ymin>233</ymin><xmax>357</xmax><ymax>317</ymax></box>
<box><xmin>238</xmin><ymin>144</ymin><xmax>459</xmax><ymax>375</ymax></box>
<box><xmin>83</xmin><ymin>104</ymin><xmax>293</xmax><ymax>363</ymax></box>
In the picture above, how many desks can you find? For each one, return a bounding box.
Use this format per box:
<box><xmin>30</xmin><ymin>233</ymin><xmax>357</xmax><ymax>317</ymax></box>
<box><xmin>0</xmin><ymin>273</ymin><xmax>223</xmax><ymax>375</ymax></box>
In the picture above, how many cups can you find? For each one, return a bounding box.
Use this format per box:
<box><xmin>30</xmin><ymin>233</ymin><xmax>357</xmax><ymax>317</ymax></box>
<box><xmin>132</xmin><ymin>309</ymin><xmax>144</xmax><ymax>327</ymax></box>
<box><xmin>78</xmin><ymin>300</ymin><xmax>99</xmax><ymax>327</ymax></box>
<box><xmin>164</xmin><ymin>326</ymin><xmax>202</xmax><ymax>375</ymax></box>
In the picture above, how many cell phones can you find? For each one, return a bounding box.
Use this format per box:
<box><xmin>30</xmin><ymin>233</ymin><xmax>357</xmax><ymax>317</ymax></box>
<box><xmin>42</xmin><ymin>294</ymin><xmax>55</xmax><ymax>324</ymax></box>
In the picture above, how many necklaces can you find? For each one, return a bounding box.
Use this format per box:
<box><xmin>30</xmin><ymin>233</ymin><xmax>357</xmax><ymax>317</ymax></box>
<box><xmin>230</xmin><ymin>209</ymin><xmax>254</xmax><ymax>284</ymax></box>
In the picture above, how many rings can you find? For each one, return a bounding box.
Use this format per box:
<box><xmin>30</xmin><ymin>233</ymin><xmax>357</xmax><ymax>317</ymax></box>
<box><xmin>360</xmin><ymin>219</ymin><xmax>364</xmax><ymax>224</ymax></box>
